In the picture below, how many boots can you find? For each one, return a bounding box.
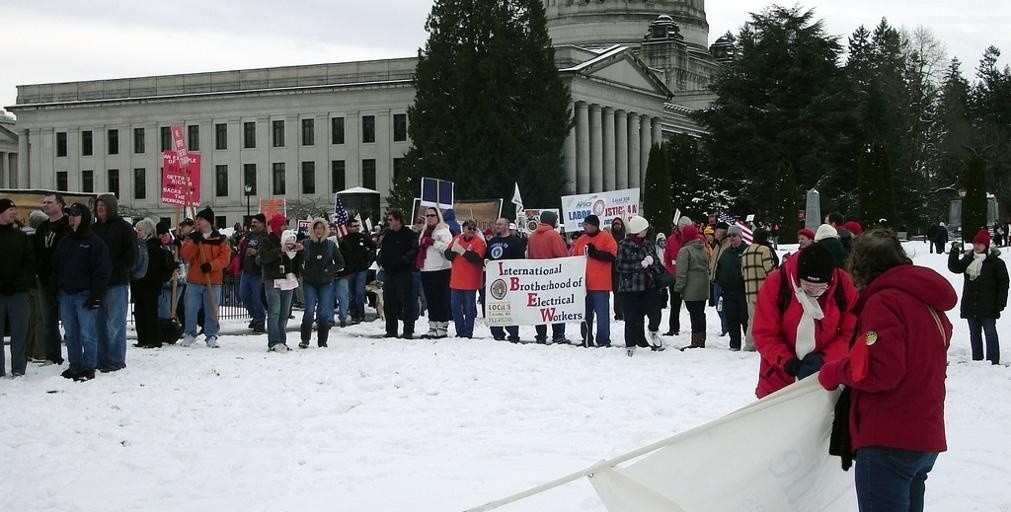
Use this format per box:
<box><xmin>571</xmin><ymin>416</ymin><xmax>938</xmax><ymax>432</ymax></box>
<box><xmin>420</xmin><ymin>319</ymin><xmax>450</xmax><ymax>338</ymax></box>
<box><xmin>680</xmin><ymin>331</ymin><xmax>707</xmax><ymax>350</ymax></box>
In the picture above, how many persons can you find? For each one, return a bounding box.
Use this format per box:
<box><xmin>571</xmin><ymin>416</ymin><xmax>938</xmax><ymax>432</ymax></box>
<box><xmin>946</xmin><ymin>229</ymin><xmax>1010</xmax><ymax>366</ymax></box>
<box><xmin>0</xmin><ymin>194</ymin><xmax>862</xmax><ymax>383</ymax></box>
<box><xmin>818</xmin><ymin>226</ymin><xmax>957</xmax><ymax>512</ymax></box>
<box><xmin>923</xmin><ymin>219</ymin><xmax>949</xmax><ymax>255</ymax></box>
<box><xmin>991</xmin><ymin>218</ymin><xmax>1009</xmax><ymax>248</ymax></box>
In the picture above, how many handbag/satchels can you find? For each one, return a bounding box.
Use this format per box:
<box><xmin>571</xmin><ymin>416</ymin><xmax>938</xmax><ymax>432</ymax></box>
<box><xmin>649</xmin><ymin>260</ymin><xmax>674</xmax><ymax>290</ymax></box>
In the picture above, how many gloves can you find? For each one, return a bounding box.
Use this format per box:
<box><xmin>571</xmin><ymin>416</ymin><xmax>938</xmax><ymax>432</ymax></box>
<box><xmin>82</xmin><ymin>290</ymin><xmax>106</xmax><ymax>311</ymax></box>
<box><xmin>586</xmin><ymin>243</ymin><xmax>596</xmax><ymax>259</ymax></box>
<box><xmin>641</xmin><ymin>255</ymin><xmax>653</xmax><ymax>269</ymax></box>
<box><xmin>786</xmin><ymin>351</ymin><xmax>823</xmax><ymax>380</ymax></box>
<box><xmin>452</xmin><ymin>242</ymin><xmax>465</xmax><ymax>256</ymax></box>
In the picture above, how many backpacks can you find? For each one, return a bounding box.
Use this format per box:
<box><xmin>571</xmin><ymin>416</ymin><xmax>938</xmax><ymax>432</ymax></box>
<box><xmin>825</xmin><ymin>386</ymin><xmax>859</xmax><ymax>468</ymax></box>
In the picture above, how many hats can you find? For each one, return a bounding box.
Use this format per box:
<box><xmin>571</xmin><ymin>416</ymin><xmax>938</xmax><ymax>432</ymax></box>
<box><xmin>540</xmin><ymin>210</ymin><xmax>558</xmax><ymax>228</ymax></box>
<box><xmin>797</xmin><ymin>244</ymin><xmax>833</xmax><ymax>284</ymax></box>
<box><xmin>628</xmin><ymin>216</ymin><xmax>649</xmax><ymax>234</ymax></box>
<box><xmin>0</xmin><ymin>198</ymin><xmax>15</xmax><ymax>214</ymax></box>
<box><xmin>655</xmin><ymin>232</ymin><xmax>667</xmax><ymax>243</ymax></box>
<box><xmin>797</xmin><ymin>228</ymin><xmax>816</xmax><ymax>240</ymax></box>
<box><xmin>197</xmin><ymin>208</ymin><xmax>214</xmax><ymax>226</ymax></box>
<box><xmin>814</xmin><ymin>224</ymin><xmax>839</xmax><ymax>243</ymax></box>
<box><xmin>677</xmin><ymin>215</ymin><xmax>744</xmax><ymax>242</ymax></box>
<box><xmin>62</xmin><ymin>202</ymin><xmax>90</xmax><ymax>221</ymax></box>
<box><xmin>972</xmin><ymin>230</ymin><xmax>990</xmax><ymax>249</ymax></box>
<box><xmin>585</xmin><ymin>215</ymin><xmax>599</xmax><ymax>228</ymax></box>
<box><xmin>753</xmin><ymin>227</ymin><xmax>769</xmax><ymax>243</ymax></box>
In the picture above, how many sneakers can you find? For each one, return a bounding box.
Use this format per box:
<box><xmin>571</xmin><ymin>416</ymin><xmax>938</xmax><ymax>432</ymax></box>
<box><xmin>267</xmin><ymin>341</ymin><xmax>291</xmax><ymax>354</ymax></box>
<box><xmin>728</xmin><ymin>346</ymin><xmax>741</xmax><ymax>351</ymax></box>
<box><xmin>181</xmin><ymin>334</ymin><xmax>196</xmax><ymax>346</ymax></box>
<box><xmin>327</xmin><ymin>318</ymin><xmax>367</xmax><ymax>330</ymax></box>
<box><xmin>381</xmin><ymin>331</ymin><xmax>398</xmax><ymax>340</ymax></box>
<box><xmin>1</xmin><ymin>351</ymin><xmax>62</xmax><ymax>378</ymax></box>
<box><xmin>317</xmin><ymin>341</ymin><xmax>328</xmax><ymax>349</ymax></box>
<box><xmin>96</xmin><ymin>362</ymin><xmax>125</xmax><ymax>373</ymax></box>
<box><xmin>297</xmin><ymin>339</ymin><xmax>309</xmax><ymax>348</ymax></box>
<box><xmin>454</xmin><ymin>328</ymin><xmax>665</xmax><ymax>351</ymax></box>
<box><xmin>206</xmin><ymin>335</ymin><xmax>219</xmax><ymax>349</ymax></box>
<box><xmin>134</xmin><ymin>339</ymin><xmax>162</xmax><ymax>349</ymax></box>
<box><xmin>61</xmin><ymin>366</ymin><xmax>95</xmax><ymax>382</ymax></box>
<box><xmin>248</xmin><ymin>318</ymin><xmax>265</xmax><ymax>333</ymax></box>
<box><xmin>400</xmin><ymin>333</ymin><xmax>412</xmax><ymax>340</ymax></box>
